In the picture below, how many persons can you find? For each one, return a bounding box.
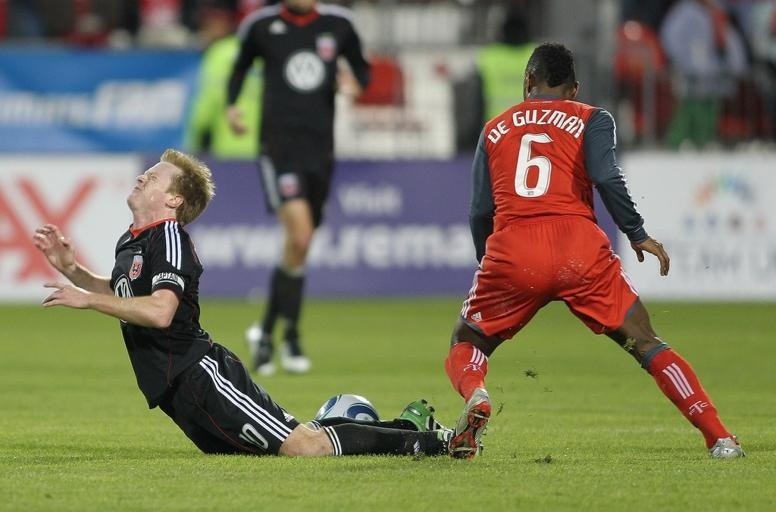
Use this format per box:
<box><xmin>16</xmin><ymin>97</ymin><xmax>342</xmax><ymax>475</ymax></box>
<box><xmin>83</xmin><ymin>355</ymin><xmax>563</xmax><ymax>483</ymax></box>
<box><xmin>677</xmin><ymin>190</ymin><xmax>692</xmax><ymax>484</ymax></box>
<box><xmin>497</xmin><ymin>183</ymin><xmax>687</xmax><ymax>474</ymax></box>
<box><xmin>34</xmin><ymin>150</ymin><xmax>482</xmax><ymax>458</ymax></box>
<box><xmin>1</xmin><ymin>0</ymin><xmax>776</xmax><ymax>158</ymax></box>
<box><xmin>226</xmin><ymin>0</ymin><xmax>372</xmax><ymax>375</ymax></box>
<box><xmin>446</xmin><ymin>44</ymin><xmax>744</xmax><ymax>458</ymax></box>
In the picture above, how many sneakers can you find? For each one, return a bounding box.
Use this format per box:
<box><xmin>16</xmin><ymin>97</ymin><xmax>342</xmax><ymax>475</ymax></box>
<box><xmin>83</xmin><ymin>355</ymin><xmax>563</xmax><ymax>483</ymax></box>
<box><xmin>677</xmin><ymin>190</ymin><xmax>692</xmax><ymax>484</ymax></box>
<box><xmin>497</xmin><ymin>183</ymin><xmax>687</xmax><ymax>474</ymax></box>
<box><xmin>447</xmin><ymin>391</ymin><xmax>492</xmax><ymax>459</ymax></box>
<box><xmin>707</xmin><ymin>436</ymin><xmax>746</xmax><ymax>459</ymax></box>
<box><xmin>281</xmin><ymin>331</ymin><xmax>310</xmax><ymax>371</ymax></box>
<box><xmin>418</xmin><ymin>429</ymin><xmax>453</xmax><ymax>457</ymax></box>
<box><xmin>246</xmin><ymin>327</ymin><xmax>275</xmax><ymax>378</ymax></box>
<box><xmin>403</xmin><ymin>400</ymin><xmax>444</xmax><ymax>430</ymax></box>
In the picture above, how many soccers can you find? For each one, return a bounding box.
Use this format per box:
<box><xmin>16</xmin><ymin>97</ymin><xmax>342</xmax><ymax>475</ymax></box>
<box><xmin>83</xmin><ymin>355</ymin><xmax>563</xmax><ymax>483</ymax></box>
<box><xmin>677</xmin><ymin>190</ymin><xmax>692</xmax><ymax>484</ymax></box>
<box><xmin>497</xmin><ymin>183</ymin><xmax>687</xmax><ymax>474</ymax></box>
<box><xmin>314</xmin><ymin>394</ymin><xmax>379</xmax><ymax>421</ymax></box>
